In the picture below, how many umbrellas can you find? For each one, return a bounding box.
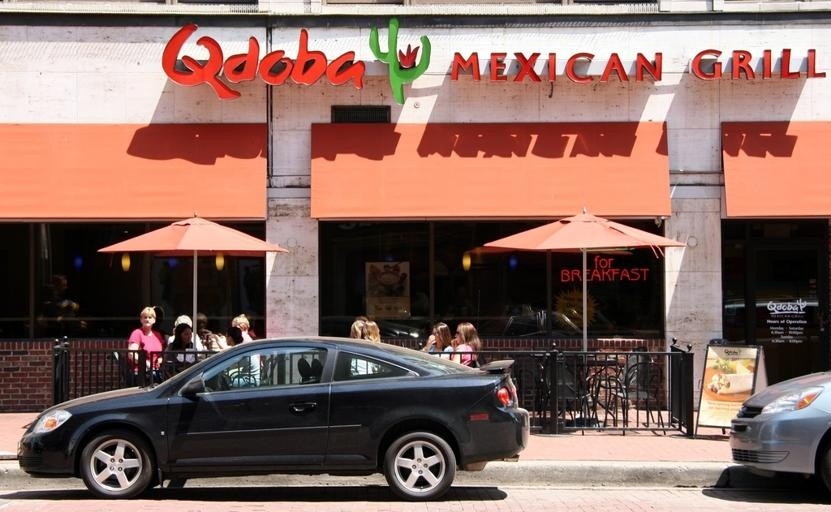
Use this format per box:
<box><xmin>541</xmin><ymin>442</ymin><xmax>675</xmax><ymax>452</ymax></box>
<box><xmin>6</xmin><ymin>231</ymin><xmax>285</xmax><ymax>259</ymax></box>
<box><xmin>483</xmin><ymin>205</ymin><xmax>686</xmax><ymax>378</ymax></box>
<box><xmin>97</xmin><ymin>213</ymin><xmax>289</xmax><ymax>350</ymax></box>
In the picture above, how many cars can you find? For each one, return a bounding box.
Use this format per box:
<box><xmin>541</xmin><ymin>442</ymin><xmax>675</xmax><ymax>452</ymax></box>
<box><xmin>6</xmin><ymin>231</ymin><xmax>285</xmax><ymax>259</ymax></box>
<box><xmin>17</xmin><ymin>335</ymin><xmax>531</xmax><ymax>500</ymax></box>
<box><xmin>729</xmin><ymin>370</ymin><xmax>830</xmax><ymax>495</ymax></box>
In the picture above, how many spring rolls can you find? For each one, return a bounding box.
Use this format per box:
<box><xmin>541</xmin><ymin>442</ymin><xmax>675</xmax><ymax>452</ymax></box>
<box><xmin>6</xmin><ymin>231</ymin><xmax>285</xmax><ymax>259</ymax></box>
<box><xmin>707</xmin><ymin>372</ymin><xmax>755</xmax><ymax>395</ymax></box>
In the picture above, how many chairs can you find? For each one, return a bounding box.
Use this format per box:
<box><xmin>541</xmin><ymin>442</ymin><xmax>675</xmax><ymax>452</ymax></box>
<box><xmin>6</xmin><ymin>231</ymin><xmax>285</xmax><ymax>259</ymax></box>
<box><xmin>468</xmin><ymin>348</ymin><xmax>668</xmax><ymax>437</ymax></box>
<box><xmin>298</xmin><ymin>358</ymin><xmax>319</xmax><ymax>384</ymax></box>
<box><xmin>114</xmin><ymin>348</ymin><xmax>150</xmax><ymax>388</ymax></box>
<box><xmin>154</xmin><ymin>352</ymin><xmax>165</xmax><ymax>384</ymax></box>
<box><xmin>310</xmin><ymin>359</ymin><xmax>323</xmax><ymax>377</ymax></box>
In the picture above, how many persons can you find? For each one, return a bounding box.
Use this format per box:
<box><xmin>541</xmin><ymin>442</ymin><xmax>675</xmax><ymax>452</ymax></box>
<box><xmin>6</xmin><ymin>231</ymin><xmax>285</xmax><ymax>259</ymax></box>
<box><xmin>350</xmin><ymin>316</ymin><xmax>482</xmax><ymax>375</ymax></box>
<box><xmin>127</xmin><ymin>306</ymin><xmax>262</xmax><ymax>388</ymax></box>
<box><xmin>38</xmin><ymin>275</ymin><xmax>87</xmax><ymax>339</ymax></box>
